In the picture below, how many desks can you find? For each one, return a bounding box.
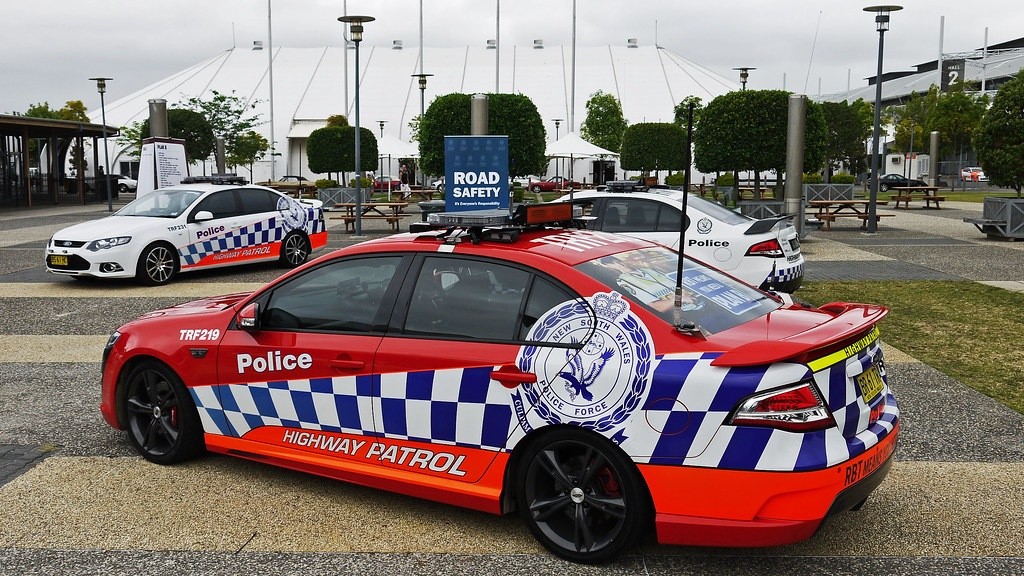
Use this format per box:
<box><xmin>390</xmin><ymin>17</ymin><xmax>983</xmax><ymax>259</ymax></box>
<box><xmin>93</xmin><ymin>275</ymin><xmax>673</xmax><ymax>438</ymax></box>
<box><xmin>334</xmin><ymin>203</ymin><xmax>408</xmax><ymax>232</ymax></box>
<box><xmin>738</xmin><ymin>187</ymin><xmax>768</xmax><ymax>198</ymax></box>
<box><xmin>893</xmin><ymin>187</ymin><xmax>943</xmax><ymax>209</ymax></box>
<box><xmin>392</xmin><ymin>190</ymin><xmax>438</xmax><ymax>201</ymax></box>
<box><xmin>809</xmin><ymin>201</ymin><xmax>888</xmax><ymax>232</ymax></box>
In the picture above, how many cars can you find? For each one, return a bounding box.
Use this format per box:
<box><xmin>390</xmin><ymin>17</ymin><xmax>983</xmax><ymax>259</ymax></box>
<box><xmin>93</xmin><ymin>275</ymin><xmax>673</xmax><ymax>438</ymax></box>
<box><xmin>29</xmin><ymin>167</ymin><xmax>37</xmax><ymax>176</ymax></box>
<box><xmin>960</xmin><ymin>167</ymin><xmax>990</xmax><ymax>182</ymax></box>
<box><xmin>431</xmin><ymin>177</ymin><xmax>445</xmax><ymax>195</ymax></box>
<box><xmin>44</xmin><ymin>172</ymin><xmax>329</xmax><ymax>288</ymax></box>
<box><xmin>97</xmin><ymin>200</ymin><xmax>909</xmax><ymax>562</ymax></box>
<box><xmin>526</xmin><ymin>175</ymin><xmax>582</xmax><ymax>193</ymax></box>
<box><xmin>865</xmin><ymin>173</ymin><xmax>929</xmax><ymax>192</ymax></box>
<box><xmin>541</xmin><ymin>177</ymin><xmax>807</xmax><ymax>298</ymax></box>
<box><xmin>515</xmin><ymin>175</ymin><xmax>540</xmax><ymax>188</ymax></box>
<box><xmin>106</xmin><ymin>173</ymin><xmax>137</xmax><ymax>194</ymax></box>
<box><xmin>278</xmin><ymin>175</ymin><xmax>318</xmax><ymax>194</ymax></box>
<box><xmin>370</xmin><ymin>175</ymin><xmax>402</xmax><ymax>191</ymax></box>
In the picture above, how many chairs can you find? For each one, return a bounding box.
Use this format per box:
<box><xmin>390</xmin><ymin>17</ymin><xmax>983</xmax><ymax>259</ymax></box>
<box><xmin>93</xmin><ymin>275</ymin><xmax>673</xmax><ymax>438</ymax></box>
<box><xmin>629</xmin><ymin>207</ymin><xmax>646</xmax><ymax>224</ymax></box>
<box><xmin>185</xmin><ymin>194</ymin><xmax>197</xmax><ymax>205</ymax></box>
<box><xmin>605</xmin><ymin>207</ymin><xmax>620</xmax><ymax>225</ymax></box>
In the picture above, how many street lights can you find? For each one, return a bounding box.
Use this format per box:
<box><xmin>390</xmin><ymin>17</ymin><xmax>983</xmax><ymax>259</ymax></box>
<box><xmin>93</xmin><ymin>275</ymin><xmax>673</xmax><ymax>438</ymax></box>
<box><xmin>376</xmin><ymin>120</ymin><xmax>389</xmax><ymax>143</ymax></box>
<box><xmin>337</xmin><ymin>15</ymin><xmax>378</xmax><ymax>236</ymax></box>
<box><xmin>411</xmin><ymin>72</ymin><xmax>435</xmax><ymax>191</ymax></box>
<box><xmin>550</xmin><ymin>118</ymin><xmax>565</xmax><ymax>193</ymax></box>
<box><xmin>731</xmin><ymin>65</ymin><xmax>757</xmax><ymax>209</ymax></box>
<box><xmin>862</xmin><ymin>3</ymin><xmax>904</xmax><ymax>234</ymax></box>
<box><xmin>85</xmin><ymin>77</ymin><xmax>117</xmax><ymax>212</ymax></box>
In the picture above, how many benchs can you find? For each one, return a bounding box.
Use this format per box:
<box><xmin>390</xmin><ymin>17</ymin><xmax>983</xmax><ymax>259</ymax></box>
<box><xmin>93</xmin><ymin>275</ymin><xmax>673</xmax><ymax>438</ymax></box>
<box><xmin>804</xmin><ymin>212</ymin><xmax>896</xmax><ymax>222</ymax></box>
<box><xmin>889</xmin><ymin>194</ymin><xmax>948</xmax><ymax>201</ymax></box>
<box><xmin>963</xmin><ymin>217</ymin><xmax>1006</xmax><ymax>237</ymax></box>
<box><xmin>329</xmin><ymin>214</ymin><xmax>412</xmax><ymax>224</ymax></box>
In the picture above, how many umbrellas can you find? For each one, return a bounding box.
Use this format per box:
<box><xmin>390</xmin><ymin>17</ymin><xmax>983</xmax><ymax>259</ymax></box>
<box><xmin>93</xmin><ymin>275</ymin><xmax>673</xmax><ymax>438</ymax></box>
<box><xmin>549</xmin><ymin>130</ymin><xmax>620</xmax><ymax>217</ymax></box>
<box><xmin>378</xmin><ymin>133</ymin><xmax>422</xmax><ymax>202</ymax></box>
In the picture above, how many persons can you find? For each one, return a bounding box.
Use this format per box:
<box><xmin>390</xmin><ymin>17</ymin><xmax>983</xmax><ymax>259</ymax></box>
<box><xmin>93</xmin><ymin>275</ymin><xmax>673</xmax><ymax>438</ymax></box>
<box><xmin>596</xmin><ymin>254</ymin><xmax>698</xmax><ymax>317</ymax></box>
<box><xmin>399</xmin><ymin>167</ymin><xmax>405</xmax><ymax>191</ymax></box>
<box><xmin>402</xmin><ymin>165</ymin><xmax>411</xmax><ymax>199</ymax></box>
